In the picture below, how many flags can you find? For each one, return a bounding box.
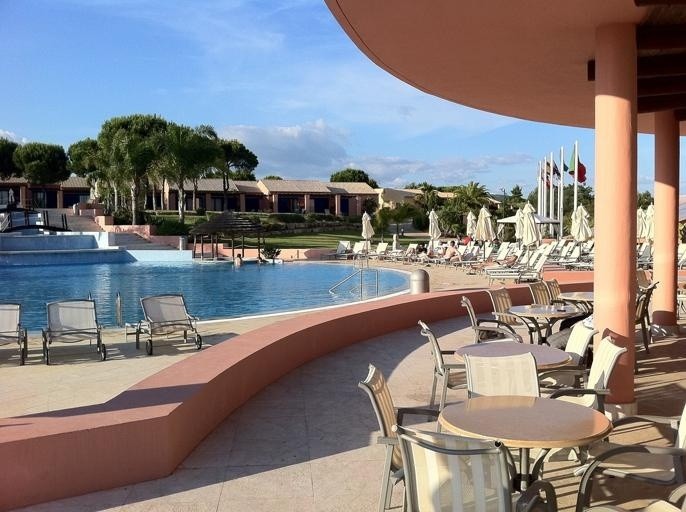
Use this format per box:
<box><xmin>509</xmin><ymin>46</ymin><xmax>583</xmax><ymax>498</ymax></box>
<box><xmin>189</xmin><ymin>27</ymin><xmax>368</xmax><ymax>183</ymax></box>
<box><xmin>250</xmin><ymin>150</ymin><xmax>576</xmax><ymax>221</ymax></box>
<box><xmin>541</xmin><ymin>154</ymin><xmax>587</xmax><ymax>189</ymax></box>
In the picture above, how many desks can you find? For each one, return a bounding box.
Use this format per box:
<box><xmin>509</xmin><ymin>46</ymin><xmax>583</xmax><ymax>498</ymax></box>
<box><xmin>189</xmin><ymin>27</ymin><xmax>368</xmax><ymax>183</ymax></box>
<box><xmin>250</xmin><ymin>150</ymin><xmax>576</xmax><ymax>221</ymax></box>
<box><xmin>556</xmin><ymin>290</ymin><xmax>598</xmax><ymax>308</ymax></box>
<box><xmin>458</xmin><ymin>336</ymin><xmax>572</xmax><ymax>372</ymax></box>
<box><xmin>511</xmin><ymin>302</ymin><xmax>579</xmax><ymax>332</ymax></box>
<box><xmin>445</xmin><ymin>396</ymin><xmax>615</xmax><ymax>472</ymax></box>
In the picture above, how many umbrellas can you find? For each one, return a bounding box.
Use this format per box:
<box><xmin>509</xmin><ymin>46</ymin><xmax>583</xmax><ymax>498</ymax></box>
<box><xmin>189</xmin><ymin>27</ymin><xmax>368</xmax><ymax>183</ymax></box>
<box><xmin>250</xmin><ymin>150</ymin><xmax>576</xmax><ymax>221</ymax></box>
<box><xmin>361</xmin><ymin>210</ymin><xmax>374</xmax><ymax>252</ymax></box>
<box><xmin>428</xmin><ymin>199</ymin><xmax>654</xmax><ymax>266</ymax></box>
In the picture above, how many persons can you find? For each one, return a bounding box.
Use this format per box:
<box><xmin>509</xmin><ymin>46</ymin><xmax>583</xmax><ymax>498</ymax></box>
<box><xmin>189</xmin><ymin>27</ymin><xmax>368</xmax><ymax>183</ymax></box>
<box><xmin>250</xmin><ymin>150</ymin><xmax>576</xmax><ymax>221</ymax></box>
<box><xmin>401</xmin><ymin>227</ymin><xmax>404</xmax><ymax>236</ymax></box>
<box><xmin>406</xmin><ymin>242</ymin><xmax>427</xmax><ymax>256</ymax></box>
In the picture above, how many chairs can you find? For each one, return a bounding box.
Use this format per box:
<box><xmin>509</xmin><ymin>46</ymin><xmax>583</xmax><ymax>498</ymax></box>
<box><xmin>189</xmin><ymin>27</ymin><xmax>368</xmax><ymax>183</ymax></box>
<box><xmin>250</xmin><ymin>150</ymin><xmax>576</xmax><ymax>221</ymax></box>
<box><xmin>416</xmin><ymin>319</ymin><xmax>465</xmax><ymax>400</ymax></box>
<box><xmin>356</xmin><ymin>366</ymin><xmax>411</xmax><ymax>505</ymax></box>
<box><xmin>394</xmin><ymin>420</ymin><xmax>560</xmax><ymax>512</ymax></box>
<box><xmin>320</xmin><ymin>236</ymin><xmax>686</xmax><ymax>288</ymax></box>
<box><xmin>561</xmin><ymin>337</ymin><xmax>628</xmax><ymax>414</ymax></box>
<box><xmin>527</xmin><ymin>283</ymin><xmax>567</xmax><ymax>308</ymax></box>
<box><xmin>460</xmin><ymin>353</ymin><xmax>547</xmax><ymax>402</ymax></box>
<box><xmin>559</xmin><ymin>323</ymin><xmax>593</xmax><ymax>384</ymax></box>
<box><xmin>488</xmin><ymin>288</ymin><xmax>526</xmax><ymax>333</ymax></box>
<box><xmin>575</xmin><ymin>414</ymin><xmax>686</xmax><ymax>512</ymax></box>
<box><xmin>632</xmin><ymin>282</ymin><xmax>659</xmax><ymax>355</ymax></box>
<box><xmin>0</xmin><ymin>303</ymin><xmax>28</xmax><ymax>366</ymax></box>
<box><xmin>457</xmin><ymin>298</ymin><xmax>523</xmax><ymax>343</ymax></box>
<box><xmin>124</xmin><ymin>292</ymin><xmax>202</xmax><ymax>356</ymax></box>
<box><xmin>42</xmin><ymin>298</ymin><xmax>107</xmax><ymax>361</ymax></box>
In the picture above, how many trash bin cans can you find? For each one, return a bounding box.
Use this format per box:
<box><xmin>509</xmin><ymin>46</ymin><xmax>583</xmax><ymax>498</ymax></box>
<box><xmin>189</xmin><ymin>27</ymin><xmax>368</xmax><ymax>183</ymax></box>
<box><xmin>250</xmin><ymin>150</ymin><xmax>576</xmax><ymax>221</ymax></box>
<box><xmin>179</xmin><ymin>235</ymin><xmax>188</xmax><ymax>250</ymax></box>
<box><xmin>73</xmin><ymin>204</ymin><xmax>78</xmax><ymax>215</ymax></box>
